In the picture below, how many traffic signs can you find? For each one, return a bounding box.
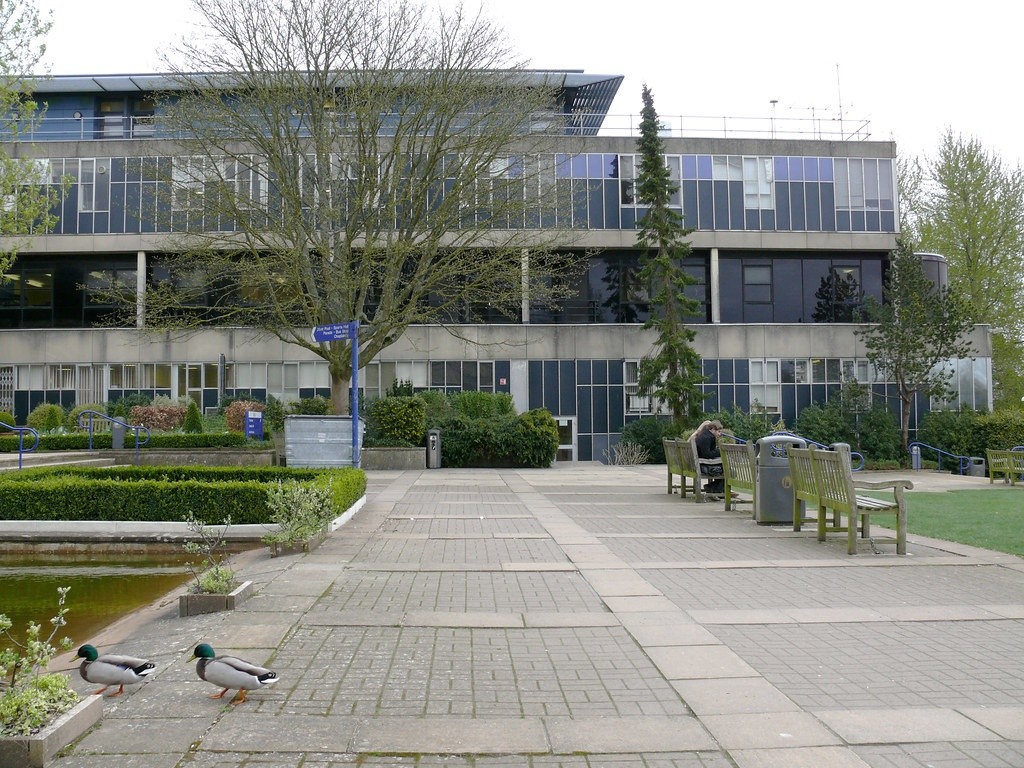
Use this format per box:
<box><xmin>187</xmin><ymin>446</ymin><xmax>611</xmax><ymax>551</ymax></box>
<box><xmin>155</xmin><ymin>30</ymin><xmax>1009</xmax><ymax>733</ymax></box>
<box><xmin>309</xmin><ymin>321</ymin><xmax>354</xmax><ymax>343</ymax></box>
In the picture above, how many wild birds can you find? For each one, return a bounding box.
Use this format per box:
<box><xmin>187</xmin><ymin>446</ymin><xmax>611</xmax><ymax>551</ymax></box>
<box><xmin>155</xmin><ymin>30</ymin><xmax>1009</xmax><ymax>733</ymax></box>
<box><xmin>186</xmin><ymin>643</ymin><xmax>280</xmax><ymax>705</ymax></box>
<box><xmin>69</xmin><ymin>644</ymin><xmax>158</xmax><ymax>697</ymax></box>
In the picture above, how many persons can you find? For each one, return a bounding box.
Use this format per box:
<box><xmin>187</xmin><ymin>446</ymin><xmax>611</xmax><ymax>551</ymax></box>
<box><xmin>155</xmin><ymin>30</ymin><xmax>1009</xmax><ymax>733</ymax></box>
<box><xmin>686</xmin><ymin>419</ymin><xmax>739</xmax><ymax>498</ymax></box>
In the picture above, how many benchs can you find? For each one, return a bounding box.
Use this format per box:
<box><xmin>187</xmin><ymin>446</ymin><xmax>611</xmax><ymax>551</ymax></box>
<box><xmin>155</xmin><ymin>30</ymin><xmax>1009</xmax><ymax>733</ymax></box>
<box><xmin>986</xmin><ymin>448</ymin><xmax>1024</xmax><ymax>486</ymax></box>
<box><xmin>663</xmin><ymin>437</ymin><xmax>739</xmax><ymax>504</ymax></box>
<box><xmin>786</xmin><ymin>442</ymin><xmax>913</xmax><ymax>555</ymax></box>
<box><xmin>719</xmin><ymin>439</ymin><xmax>760</xmax><ymax>520</ymax></box>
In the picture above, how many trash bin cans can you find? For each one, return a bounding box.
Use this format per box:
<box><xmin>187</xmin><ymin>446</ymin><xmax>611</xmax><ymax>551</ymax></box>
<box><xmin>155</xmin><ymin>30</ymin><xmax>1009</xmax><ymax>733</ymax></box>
<box><xmin>426</xmin><ymin>429</ymin><xmax>441</xmax><ymax>469</ymax></box>
<box><xmin>829</xmin><ymin>443</ymin><xmax>851</xmax><ymax>472</ymax></box>
<box><xmin>112</xmin><ymin>416</ymin><xmax>126</xmax><ymax>448</ymax></box>
<box><xmin>753</xmin><ymin>436</ymin><xmax>806</xmax><ymax>526</ymax></box>
<box><xmin>967</xmin><ymin>457</ymin><xmax>986</xmax><ymax>477</ymax></box>
